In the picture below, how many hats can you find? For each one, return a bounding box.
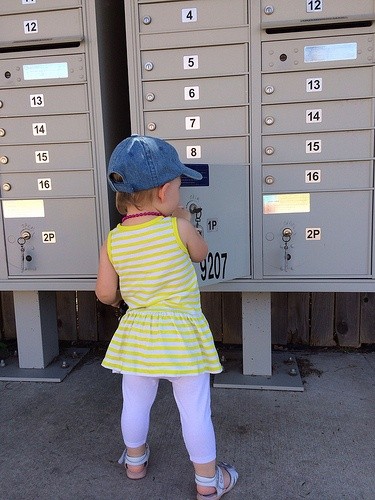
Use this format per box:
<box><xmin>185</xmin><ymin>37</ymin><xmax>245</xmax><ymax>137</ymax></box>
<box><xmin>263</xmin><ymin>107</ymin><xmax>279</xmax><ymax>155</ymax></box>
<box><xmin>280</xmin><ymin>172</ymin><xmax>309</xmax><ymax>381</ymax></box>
<box><xmin>106</xmin><ymin>134</ymin><xmax>203</xmax><ymax>192</ymax></box>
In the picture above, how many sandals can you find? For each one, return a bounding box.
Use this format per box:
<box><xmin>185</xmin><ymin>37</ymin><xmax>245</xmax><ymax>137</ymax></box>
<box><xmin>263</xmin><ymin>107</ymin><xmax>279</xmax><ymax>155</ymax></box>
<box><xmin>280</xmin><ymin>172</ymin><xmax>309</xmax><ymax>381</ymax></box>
<box><xmin>117</xmin><ymin>442</ymin><xmax>150</xmax><ymax>480</ymax></box>
<box><xmin>194</xmin><ymin>461</ymin><xmax>239</xmax><ymax>499</ymax></box>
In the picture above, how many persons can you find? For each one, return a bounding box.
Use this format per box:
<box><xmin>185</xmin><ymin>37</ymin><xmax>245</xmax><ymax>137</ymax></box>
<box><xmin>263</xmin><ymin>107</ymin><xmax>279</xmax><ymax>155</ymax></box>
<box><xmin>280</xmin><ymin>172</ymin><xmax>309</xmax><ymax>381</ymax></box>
<box><xmin>93</xmin><ymin>135</ymin><xmax>240</xmax><ymax>500</ymax></box>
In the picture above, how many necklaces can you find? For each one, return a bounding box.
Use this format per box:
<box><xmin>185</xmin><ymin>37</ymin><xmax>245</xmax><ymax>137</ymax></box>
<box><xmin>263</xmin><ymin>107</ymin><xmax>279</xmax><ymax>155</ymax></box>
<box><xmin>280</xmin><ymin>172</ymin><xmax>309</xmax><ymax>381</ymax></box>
<box><xmin>121</xmin><ymin>211</ymin><xmax>166</xmax><ymax>224</ymax></box>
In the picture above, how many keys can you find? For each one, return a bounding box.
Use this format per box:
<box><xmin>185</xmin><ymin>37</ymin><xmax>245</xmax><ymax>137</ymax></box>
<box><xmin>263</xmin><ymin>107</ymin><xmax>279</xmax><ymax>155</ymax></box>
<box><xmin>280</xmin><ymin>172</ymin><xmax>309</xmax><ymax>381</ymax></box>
<box><xmin>284</xmin><ymin>245</ymin><xmax>289</xmax><ymax>263</ymax></box>
<box><xmin>194</xmin><ymin>217</ymin><xmax>201</xmax><ymax>228</ymax></box>
<box><xmin>17</xmin><ymin>246</ymin><xmax>26</xmax><ymax>260</ymax></box>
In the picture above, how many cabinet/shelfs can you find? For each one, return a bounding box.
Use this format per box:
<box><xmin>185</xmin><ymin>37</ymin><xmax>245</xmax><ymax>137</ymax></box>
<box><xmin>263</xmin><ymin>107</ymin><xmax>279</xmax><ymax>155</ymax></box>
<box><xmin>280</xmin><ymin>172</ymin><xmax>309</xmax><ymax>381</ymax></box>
<box><xmin>115</xmin><ymin>0</ymin><xmax>375</xmax><ymax>393</ymax></box>
<box><xmin>0</xmin><ymin>0</ymin><xmax>133</xmax><ymax>382</ymax></box>
